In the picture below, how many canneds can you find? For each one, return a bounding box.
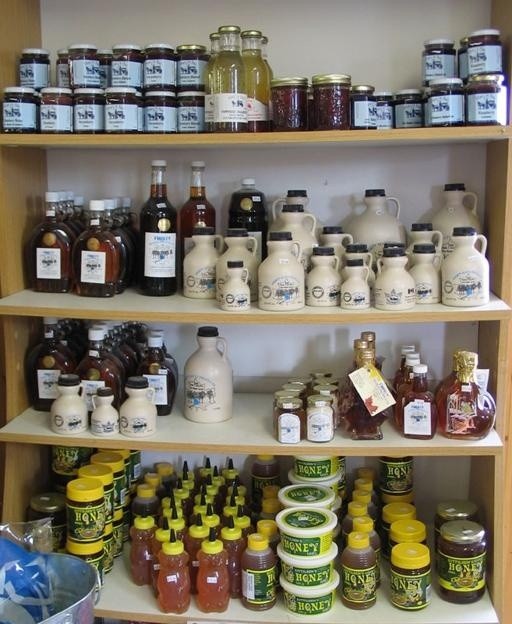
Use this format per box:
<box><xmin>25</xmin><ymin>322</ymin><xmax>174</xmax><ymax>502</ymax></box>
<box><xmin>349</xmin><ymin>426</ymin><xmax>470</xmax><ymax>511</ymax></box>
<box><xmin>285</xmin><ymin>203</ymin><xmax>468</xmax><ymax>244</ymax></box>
<box><xmin>22</xmin><ymin>493</ymin><xmax>66</xmax><ymax>552</ymax></box>
<box><xmin>433</xmin><ymin>498</ymin><xmax>478</xmax><ymax>560</ymax></box>
<box><xmin>273</xmin><ymin>371</ymin><xmax>339</xmax><ymax>443</ymax></box>
<box><xmin>434</xmin><ymin>518</ymin><xmax>487</xmax><ymax>602</ymax></box>
<box><xmin>3</xmin><ymin>43</ymin><xmax>209</xmax><ymax>132</ymax></box>
<box><xmin>351</xmin><ymin>27</ymin><xmax>507</xmax><ymax>130</ymax></box>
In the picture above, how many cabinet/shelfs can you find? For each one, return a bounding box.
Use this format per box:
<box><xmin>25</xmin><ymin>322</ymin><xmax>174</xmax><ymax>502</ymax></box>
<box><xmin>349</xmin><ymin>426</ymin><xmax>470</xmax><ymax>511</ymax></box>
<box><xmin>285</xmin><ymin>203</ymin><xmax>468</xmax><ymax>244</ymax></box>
<box><xmin>1</xmin><ymin>0</ymin><xmax>512</xmax><ymax>624</ymax></box>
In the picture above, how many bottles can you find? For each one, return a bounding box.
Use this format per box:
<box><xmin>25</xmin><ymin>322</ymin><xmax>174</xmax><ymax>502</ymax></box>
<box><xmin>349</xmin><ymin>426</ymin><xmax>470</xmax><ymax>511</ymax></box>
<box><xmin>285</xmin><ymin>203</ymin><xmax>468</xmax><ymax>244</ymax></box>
<box><xmin>341</xmin><ymin>456</ymin><xmax>383</xmax><ymax>612</ymax></box>
<box><xmin>182</xmin><ymin>325</ymin><xmax>235</xmax><ymax>424</ymax></box>
<box><xmin>22</xmin><ymin>315</ymin><xmax>179</xmax><ymax>438</ymax></box>
<box><xmin>126</xmin><ymin>448</ymin><xmax>284</xmax><ymax>614</ymax></box>
<box><xmin>26</xmin><ymin>158</ymin><xmax>492</xmax><ymax>311</ymax></box>
<box><xmin>203</xmin><ymin>25</ymin><xmax>275</xmax><ymax>134</ymax></box>
<box><xmin>273</xmin><ymin>330</ymin><xmax>498</xmax><ymax>444</ymax></box>
